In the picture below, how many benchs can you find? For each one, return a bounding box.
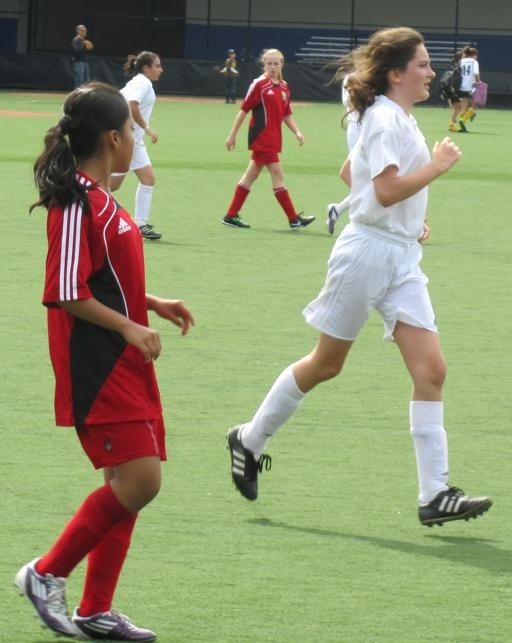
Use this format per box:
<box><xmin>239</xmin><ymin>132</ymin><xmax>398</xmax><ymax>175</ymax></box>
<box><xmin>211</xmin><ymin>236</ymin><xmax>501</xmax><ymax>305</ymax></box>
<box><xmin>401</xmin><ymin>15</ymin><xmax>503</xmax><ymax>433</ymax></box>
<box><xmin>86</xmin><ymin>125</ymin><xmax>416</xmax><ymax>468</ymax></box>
<box><xmin>293</xmin><ymin>34</ymin><xmax>479</xmax><ymax>74</ymax></box>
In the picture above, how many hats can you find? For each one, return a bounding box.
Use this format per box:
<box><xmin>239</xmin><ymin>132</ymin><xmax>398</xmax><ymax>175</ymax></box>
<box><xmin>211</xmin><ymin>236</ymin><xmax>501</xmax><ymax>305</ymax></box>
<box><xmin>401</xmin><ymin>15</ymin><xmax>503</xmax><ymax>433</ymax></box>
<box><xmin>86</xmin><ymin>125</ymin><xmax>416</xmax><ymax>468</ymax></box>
<box><xmin>227</xmin><ymin>48</ymin><xmax>236</xmax><ymax>54</ymax></box>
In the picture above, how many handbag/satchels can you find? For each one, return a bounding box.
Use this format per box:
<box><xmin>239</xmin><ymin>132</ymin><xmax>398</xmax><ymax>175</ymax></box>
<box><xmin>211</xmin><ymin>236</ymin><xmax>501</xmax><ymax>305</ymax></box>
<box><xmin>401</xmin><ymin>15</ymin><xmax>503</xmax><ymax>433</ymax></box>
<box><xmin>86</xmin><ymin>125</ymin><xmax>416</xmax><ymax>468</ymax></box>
<box><xmin>472</xmin><ymin>81</ymin><xmax>489</xmax><ymax>106</ymax></box>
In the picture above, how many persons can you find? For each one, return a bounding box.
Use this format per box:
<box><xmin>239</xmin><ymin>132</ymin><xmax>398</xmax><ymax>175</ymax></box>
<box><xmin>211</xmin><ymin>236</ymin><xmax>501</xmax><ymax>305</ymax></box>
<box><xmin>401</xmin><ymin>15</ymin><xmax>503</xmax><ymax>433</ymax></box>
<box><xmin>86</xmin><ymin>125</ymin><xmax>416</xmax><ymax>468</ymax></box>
<box><xmin>10</xmin><ymin>76</ymin><xmax>197</xmax><ymax>643</ymax></box>
<box><xmin>456</xmin><ymin>47</ymin><xmax>482</xmax><ymax>132</ymax></box>
<box><xmin>219</xmin><ymin>48</ymin><xmax>241</xmax><ymax>104</ymax></box>
<box><xmin>224</xmin><ymin>22</ymin><xmax>492</xmax><ymax>528</ymax></box>
<box><xmin>69</xmin><ymin>23</ymin><xmax>94</xmax><ymax>90</ymax></box>
<box><xmin>219</xmin><ymin>46</ymin><xmax>317</xmax><ymax>233</ymax></box>
<box><xmin>325</xmin><ymin>44</ymin><xmax>370</xmax><ymax>236</ymax></box>
<box><xmin>109</xmin><ymin>49</ymin><xmax>163</xmax><ymax>240</ymax></box>
<box><xmin>441</xmin><ymin>46</ymin><xmax>471</xmax><ymax>131</ymax></box>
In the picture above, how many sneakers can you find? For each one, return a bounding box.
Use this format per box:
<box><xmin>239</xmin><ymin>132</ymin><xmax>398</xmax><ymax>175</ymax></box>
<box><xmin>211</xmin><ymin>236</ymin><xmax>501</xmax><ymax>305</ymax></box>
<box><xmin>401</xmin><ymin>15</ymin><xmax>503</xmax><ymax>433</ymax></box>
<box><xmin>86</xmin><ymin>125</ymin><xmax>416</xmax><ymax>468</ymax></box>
<box><xmin>137</xmin><ymin>224</ymin><xmax>161</xmax><ymax>241</ymax></box>
<box><xmin>68</xmin><ymin>605</ymin><xmax>155</xmax><ymax>642</ymax></box>
<box><xmin>288</xmin><ymin>212</ymin><xmax>315</xmax><ymax>230</ymax></box>
<box><xmin>448</xmin><ymin>118</ymin><xmax>469</xmax><ymax>133</ymax></box>
<box><xmin>15</xmin><ymin>557</ymin><xmax>80</xmax><ymax>637</ymax></box>
<box><xmin>226</xmin><ymin>424</ymin><xmax>261</xmax><ymax>499</ymax></box>
<box><xmin>223</xmin><ymin>214</ymin><xmax>249</xmax><ymax>230</ymax></box>
<box><xmin>326</xmin><ymin>202</ymin><xmax>343</xmax><ymax>233</ymax></box>
<box><xmin>416</xmin><ymin>484</ymin><xmax>494</xmax><ymax>528</ymax></box>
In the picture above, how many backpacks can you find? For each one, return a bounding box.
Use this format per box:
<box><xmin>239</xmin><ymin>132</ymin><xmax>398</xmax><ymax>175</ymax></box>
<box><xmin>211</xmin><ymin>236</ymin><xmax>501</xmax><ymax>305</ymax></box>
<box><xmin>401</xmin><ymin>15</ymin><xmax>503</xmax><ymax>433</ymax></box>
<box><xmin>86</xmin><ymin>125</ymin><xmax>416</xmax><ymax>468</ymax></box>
<box><xmin>438</xmin><ymin>62</ymin><xmax>463</xmax><ymax>101</ymax></box>
